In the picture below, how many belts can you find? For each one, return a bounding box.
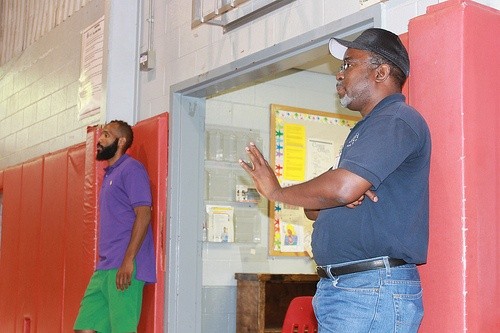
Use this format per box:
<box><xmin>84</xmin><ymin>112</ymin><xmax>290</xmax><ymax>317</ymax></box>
<box><xmin>317</xmin><ymin>259</ymin><xmax>405</xmax><ymax>278</ymax></box>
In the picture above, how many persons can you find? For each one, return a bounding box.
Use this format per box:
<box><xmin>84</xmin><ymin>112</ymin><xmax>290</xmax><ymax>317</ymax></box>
<box><xmin>239</xmin><ymin>27</ymin><xmax>431</xmax><ymax>332</ymax></box>
<box><xmin>73</xmin><ymin>120</ymin><xmax>157</xmax><ymax>333</ymax></box>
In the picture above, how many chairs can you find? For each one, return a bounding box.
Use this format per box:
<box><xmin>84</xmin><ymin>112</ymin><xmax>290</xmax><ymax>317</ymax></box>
<box><xmin>281</xmin><ymin>296</ymin><xmax>319</xmax><ymax>333</ymax></box>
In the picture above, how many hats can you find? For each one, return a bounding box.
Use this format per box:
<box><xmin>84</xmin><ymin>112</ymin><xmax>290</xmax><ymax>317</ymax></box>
<box><xmin>328</xmin><ymin>28</ymin><xmax>410</xmax><ymax>77</ymax></box>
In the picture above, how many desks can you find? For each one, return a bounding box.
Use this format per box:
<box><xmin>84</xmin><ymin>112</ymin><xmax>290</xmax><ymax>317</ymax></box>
<box><xmin>233</xmin><ymin>272</ymin><xmax>322</xmax><ymax>333</ymax></box>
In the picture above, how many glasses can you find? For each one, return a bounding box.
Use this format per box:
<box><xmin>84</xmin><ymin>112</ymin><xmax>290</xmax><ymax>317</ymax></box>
<box><xmin>340</xmin><ymin>60</ymin><xmax>380</xmax><ymax>70</ymax></box>
